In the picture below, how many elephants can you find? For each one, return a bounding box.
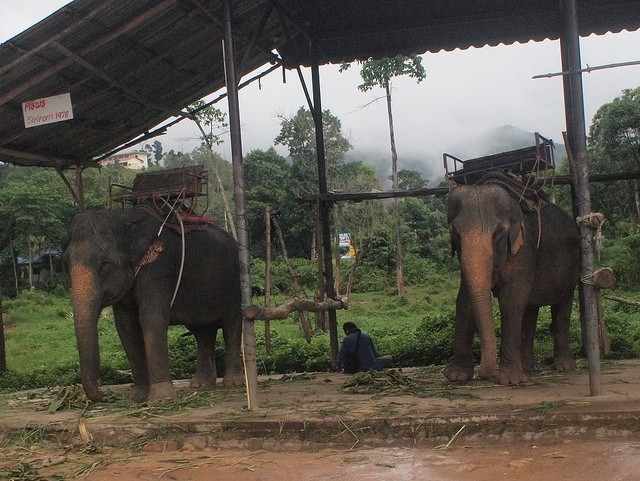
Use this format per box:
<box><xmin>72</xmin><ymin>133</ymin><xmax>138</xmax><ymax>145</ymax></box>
<box><xmin>62</xmin><ymin>205</ymin><xmax>248</xmax><ymax>403</ymax></box>
<box><xmin>442</xmin><ymin>182</ymin><xmax>580</xmax><ymax>387</ymax></box>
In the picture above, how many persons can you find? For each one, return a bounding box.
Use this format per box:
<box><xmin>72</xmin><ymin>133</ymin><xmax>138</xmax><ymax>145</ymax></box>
<box><xmin>336</xmin><ymin>321</ymin><xmax>393</xmax><ymax>374</ymax></box>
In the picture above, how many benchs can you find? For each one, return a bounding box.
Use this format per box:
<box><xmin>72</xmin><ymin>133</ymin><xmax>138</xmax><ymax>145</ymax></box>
<box><xmin>442</xmin><ymin>131</ymin><xmax>555</xmax><ymax>194</ymax></box>
<box><xmin>108</xmin><ymin>163</ymin><xmax>209</xmax><ymax>217</ymax></box>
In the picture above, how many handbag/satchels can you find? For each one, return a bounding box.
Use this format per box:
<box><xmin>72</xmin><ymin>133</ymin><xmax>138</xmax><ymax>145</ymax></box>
<box><xmin>343</xmin><ymin>351</ymin><xmax>361</xmax><ymax>374</ymax></box>
